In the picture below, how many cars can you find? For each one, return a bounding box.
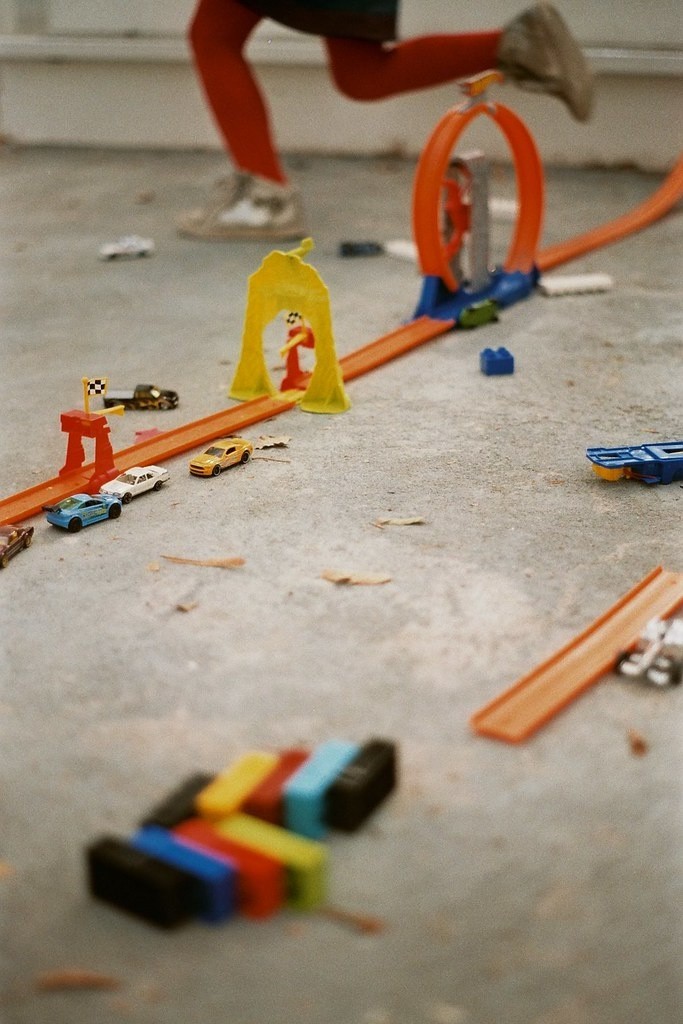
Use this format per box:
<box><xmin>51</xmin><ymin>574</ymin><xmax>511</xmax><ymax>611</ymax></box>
<box><xmin>189</xmin><ymin>437</ymin><xmax>254</xmax><ymax>478</ymax></box>
<box><xmin>98</xmin><ymin>465</ymin><xmax>171</xmax><ymax>505</ymax></box>
<box><xmin>40</xmin><ymin>493</ymin><xmax>122</xmax><ymax>532</ymax></box>
<box><xmin>0</xmin><ymin>524</ymin><xmax>35</xmax><ymax>567</ymax></box>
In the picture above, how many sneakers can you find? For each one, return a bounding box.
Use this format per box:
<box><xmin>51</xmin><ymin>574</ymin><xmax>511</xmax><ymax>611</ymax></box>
<box><xmin>178</xmin><ymin>171</ymin><xmax>307</xmax><ymax>243</ymax></box>
<box><xmin>500</xmin><ymin>3</ymin><xmax>590</xmax><ymax>123</ymax></box>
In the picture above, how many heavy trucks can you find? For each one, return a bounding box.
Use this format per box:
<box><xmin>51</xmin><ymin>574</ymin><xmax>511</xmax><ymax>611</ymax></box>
<box><xmin>104</xmin><ymin>383</ymin><xmax>178</xmax><ymax>411</ymax></box>
